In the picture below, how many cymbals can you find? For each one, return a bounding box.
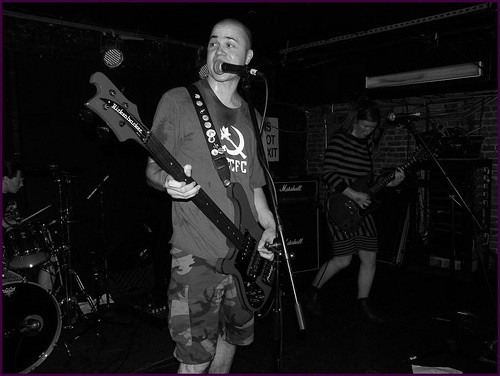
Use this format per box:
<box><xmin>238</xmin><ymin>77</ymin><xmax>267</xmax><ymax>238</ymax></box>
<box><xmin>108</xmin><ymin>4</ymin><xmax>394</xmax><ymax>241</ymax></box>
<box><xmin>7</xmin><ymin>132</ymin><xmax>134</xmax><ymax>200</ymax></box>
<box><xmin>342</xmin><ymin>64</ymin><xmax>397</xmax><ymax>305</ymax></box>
<box><xmin>36</xmin><ymin>170</ymin><xmax>89</xmax><ymax>178</ymax></box>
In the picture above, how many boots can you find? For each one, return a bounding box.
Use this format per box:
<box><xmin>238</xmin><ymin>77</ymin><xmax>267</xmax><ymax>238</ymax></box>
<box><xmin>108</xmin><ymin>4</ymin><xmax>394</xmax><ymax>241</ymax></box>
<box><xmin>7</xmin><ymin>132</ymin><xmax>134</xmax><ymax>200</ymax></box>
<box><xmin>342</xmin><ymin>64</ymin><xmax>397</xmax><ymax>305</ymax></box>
<box><xmin>357</xmin><ymin>298</ymin><xmax>388</xmax><ymax>324</ymax></box>
<box><xmin>305</xmin><ymin>286</ymin><xmax>321</xmax><ymax>315</ymax></box>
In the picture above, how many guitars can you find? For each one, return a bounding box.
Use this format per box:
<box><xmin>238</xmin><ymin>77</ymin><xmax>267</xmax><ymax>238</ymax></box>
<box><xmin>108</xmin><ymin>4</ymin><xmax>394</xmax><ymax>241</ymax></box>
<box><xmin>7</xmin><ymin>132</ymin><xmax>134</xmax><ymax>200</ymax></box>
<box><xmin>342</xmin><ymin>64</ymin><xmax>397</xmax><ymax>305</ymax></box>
<box><xmin>326</xmin><ymin>139</ymin><xmax>448</xmax><ymax>232</ymax></box>
<box><xmin>85</xmin><ymin>71</ymin><xmax>277</xmax><ymax>311</ymax></box>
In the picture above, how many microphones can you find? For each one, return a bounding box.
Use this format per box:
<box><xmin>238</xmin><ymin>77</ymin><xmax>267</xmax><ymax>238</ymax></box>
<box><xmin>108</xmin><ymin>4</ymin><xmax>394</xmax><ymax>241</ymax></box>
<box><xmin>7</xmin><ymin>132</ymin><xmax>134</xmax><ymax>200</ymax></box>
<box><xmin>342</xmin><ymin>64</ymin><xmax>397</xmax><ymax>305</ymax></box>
<box><xmin>213</xmin><ymin>60</ymin><xmax>267</xmax><ymax>80</ymax></box>
<box><xmin>388</xmin><ymin>112</ymin><xmax>420</xmax><ymax>121</ymax></box>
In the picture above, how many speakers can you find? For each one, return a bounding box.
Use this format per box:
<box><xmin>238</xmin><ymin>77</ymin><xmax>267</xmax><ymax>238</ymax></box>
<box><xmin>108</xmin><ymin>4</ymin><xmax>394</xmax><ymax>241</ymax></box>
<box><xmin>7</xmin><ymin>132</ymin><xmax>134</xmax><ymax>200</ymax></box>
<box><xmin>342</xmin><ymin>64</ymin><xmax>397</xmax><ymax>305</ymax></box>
<box><xmin>272</xmin><ymin>180</ymin><xmax>320</xmax><ymax>274</ymax></box>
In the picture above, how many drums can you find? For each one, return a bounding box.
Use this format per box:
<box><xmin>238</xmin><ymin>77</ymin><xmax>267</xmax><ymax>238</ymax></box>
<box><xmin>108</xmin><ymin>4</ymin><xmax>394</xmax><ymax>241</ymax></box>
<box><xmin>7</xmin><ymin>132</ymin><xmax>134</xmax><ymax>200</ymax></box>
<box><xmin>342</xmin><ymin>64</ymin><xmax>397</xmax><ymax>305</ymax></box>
<box><xmin>4</xmin><ymin>221</ymin><xmax>51</xmax><ymax>271</ymax></box>
<box><xmin>1</xmin><ymin>270</ymin><xmax>63</xmax><ymax>374</ymax></box>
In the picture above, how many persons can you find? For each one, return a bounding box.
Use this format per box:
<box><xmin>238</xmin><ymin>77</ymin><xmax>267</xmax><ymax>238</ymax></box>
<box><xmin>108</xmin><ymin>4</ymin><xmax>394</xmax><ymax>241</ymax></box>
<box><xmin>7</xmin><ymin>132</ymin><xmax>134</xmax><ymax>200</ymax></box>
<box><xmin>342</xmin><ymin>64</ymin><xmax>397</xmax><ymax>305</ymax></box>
<box><xmin>148</xmin><ymin>17</ymin><xmax>278</xmax><ymax>374</ymax></box>
<box><xmin>312</xmin><ymin>108</ymin><xmax>405</xmax><ymax>320</ymax></box>
<box><xmin>1</xmin><ymin>154</ymin><xmax>56</xmax><ymax>295</ymax></box>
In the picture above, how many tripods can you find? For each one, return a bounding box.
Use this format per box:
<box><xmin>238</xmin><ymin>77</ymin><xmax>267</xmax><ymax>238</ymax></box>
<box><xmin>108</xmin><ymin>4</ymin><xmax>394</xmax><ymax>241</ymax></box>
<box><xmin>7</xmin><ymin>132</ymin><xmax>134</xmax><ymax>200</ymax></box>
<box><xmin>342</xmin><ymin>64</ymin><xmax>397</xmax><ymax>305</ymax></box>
<box><xmin>36</xmin><ymin>180</ymin><xmax>126</xmax><ymax>362</ymax></box>
